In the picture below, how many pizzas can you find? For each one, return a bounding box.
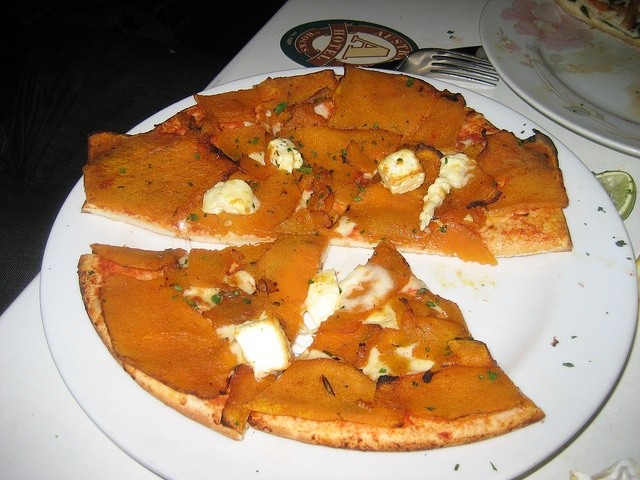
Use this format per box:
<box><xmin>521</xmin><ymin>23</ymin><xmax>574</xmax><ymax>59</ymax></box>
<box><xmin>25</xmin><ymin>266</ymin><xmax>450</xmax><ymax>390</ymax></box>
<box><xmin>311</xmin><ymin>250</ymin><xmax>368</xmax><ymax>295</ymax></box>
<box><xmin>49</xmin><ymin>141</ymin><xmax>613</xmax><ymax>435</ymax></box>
<box><xmin>244</xmin><ymin>242</ymin><xmax>545</xmax><ymax>452</ymax></box>
<box><xmin>81</xmin><ymin>65</ymin><xmax>574</xmax><ymax>266</ymax></box>
<box><xmin>78</xmin><ymin>236</ymin><xmax>331</xmax><ymax>441</ymax></box>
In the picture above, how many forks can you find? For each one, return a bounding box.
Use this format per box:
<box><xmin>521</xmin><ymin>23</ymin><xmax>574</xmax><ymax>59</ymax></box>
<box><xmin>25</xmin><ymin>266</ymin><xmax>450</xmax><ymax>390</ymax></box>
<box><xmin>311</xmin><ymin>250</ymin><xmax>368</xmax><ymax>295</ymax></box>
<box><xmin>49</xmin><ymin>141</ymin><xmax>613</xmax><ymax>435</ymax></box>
<box><xmin>392</xmin><ymin>48</ymin><xmax>500</xmax><ymax>88</ymax></box>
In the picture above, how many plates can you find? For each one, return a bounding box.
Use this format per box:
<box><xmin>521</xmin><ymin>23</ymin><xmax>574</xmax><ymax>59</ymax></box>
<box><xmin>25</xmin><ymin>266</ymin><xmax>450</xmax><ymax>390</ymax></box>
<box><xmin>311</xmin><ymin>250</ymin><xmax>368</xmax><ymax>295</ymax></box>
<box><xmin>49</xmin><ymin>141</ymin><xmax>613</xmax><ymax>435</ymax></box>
<box><xmin>39</xmin><ymin>66</ymin><xmax>639</xmax><ymax>476</ymax></box>
<box><xmin>479</xmin><ymin>2</ymin><xmax>639</xmax><ymax>158</ymax></box>
